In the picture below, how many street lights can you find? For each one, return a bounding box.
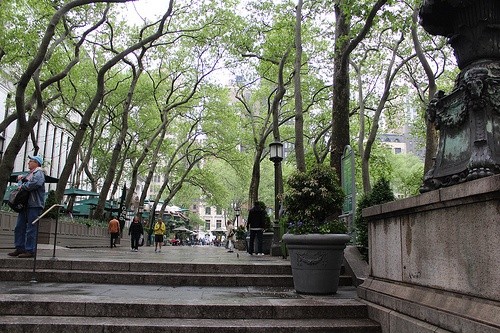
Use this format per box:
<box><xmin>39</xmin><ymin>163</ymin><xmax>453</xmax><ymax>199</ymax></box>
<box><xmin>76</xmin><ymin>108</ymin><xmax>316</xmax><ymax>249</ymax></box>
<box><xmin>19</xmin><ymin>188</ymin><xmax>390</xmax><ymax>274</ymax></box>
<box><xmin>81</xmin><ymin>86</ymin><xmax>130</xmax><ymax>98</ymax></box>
<box><xmin>269</xmin><ymin>137</ymin><xmax>286</xmax><ymax>255</ymax></box>
<box><xmin>235</xmin><ymin>207</ymin><xmax>240</xmax><ymax>228</ymax></box>
<box><xmin>138</xmin><ymin>204</ymin><xmax>144</xmax><ymax>223</ymax></box>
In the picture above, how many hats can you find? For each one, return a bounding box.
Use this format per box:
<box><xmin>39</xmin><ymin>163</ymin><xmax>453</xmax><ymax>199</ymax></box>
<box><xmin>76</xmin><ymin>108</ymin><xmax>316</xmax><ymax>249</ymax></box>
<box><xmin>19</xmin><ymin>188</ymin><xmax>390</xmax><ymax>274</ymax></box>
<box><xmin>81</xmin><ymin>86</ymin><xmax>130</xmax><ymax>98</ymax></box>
<box><xmin>28</xmin><ymin>155</ymin><xmax>42</xmax><ymax>165</ymax></box>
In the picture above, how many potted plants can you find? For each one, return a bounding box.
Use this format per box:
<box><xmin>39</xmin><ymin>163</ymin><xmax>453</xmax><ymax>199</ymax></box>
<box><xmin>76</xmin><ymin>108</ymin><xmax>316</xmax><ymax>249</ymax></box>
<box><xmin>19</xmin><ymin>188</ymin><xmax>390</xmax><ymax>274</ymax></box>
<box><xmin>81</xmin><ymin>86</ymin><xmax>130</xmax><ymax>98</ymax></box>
<box><xmin>258</xmin><ymin>201</ymin><xmax>274</xmax><ymax>253</ymax></box>
<box><xmin>275</xmin><ymin>164</ymin><xmax>352</xmax><ymax>295</ymax></box>
<box><xmin>235</xmin><ymin>225</ymin><xmax>248</xmax><ymax>251</ymax></box>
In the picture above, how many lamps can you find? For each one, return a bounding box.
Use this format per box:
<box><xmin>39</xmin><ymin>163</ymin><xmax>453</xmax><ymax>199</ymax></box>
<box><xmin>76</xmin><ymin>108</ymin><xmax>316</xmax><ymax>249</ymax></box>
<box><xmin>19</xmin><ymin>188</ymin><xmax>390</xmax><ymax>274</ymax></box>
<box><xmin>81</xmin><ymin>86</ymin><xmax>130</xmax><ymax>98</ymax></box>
<box><xmin>269</xmin><ymin>136</ymin><xmax>284</xmax><ymax>162</ymax></box>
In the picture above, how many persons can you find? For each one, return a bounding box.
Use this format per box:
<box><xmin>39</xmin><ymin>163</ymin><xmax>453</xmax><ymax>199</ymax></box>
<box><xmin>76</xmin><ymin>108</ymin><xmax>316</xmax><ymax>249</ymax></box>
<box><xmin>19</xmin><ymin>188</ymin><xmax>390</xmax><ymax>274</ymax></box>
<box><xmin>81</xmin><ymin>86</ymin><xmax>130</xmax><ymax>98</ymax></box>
<box><xmin>8</xmin><ymin>155</ymin><xmax>45</xmax><ymax>257</ymax></box>
<box><xmin>107</xmin><ymin>215</ymin><xmax>120</xmax><ymax>248</ymax></box>
<box><xmin>127</xmin><ymin>215</ymin><xmax>143</xmax><ymax>251</ymax></box>
<box><xmin>246</xmin><ymin>201</ymin><xmax>265</xmax><ymax>255</ymax></box>
<box><xmin>153</xmin><ymin>217</ymin><xmax>166</xmax><ymax>252</ymax></box>
<box><xmin>227</xmin><ymin>219</ymin><xmax>236</xmax><ymax>251</ymax></box>
<box><xmin>172</xmin><ymin>230</ymin><xmax>228</xmax><ymax>247</ymax></box>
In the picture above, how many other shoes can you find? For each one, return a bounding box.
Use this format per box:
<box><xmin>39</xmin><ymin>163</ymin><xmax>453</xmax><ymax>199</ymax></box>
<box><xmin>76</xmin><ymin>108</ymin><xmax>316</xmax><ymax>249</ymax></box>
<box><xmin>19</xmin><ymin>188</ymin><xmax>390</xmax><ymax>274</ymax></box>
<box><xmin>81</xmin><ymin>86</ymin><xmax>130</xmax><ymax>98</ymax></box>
<box><xmin>110</xmin><ymin>242</ymin><xmax>265</xmax><ymax>256</ymax></box>
<box><xmin>18</xmin><ymin>251</ymin><xmax>36</xmax><ymax>258</ymax></box>
<box><xmin>8</xmin><ymin>249</ymin><xmax>24</xmax><ymax>256</ymax></box>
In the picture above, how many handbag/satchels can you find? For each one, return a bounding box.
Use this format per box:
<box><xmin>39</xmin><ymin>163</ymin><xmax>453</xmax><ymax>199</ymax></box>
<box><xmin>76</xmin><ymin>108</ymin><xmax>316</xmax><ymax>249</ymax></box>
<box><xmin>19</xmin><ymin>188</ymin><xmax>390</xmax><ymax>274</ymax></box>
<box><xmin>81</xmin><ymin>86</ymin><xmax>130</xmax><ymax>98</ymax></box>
<box><xmin>9</xmin><ymin>185</ymin><xmax>30</xmax><ymax>212</ymax></box>
<box><xmin>228</xmin><ymin>229</ymin><xmax>235</xmax><ymax>238</ymax></box>
